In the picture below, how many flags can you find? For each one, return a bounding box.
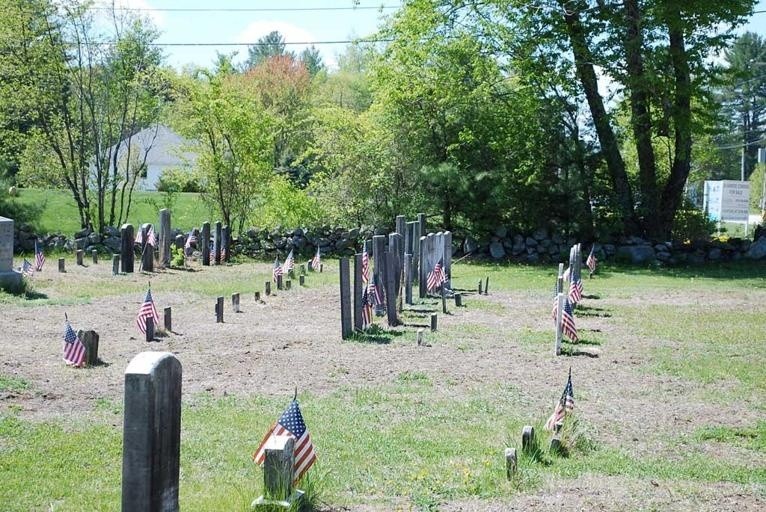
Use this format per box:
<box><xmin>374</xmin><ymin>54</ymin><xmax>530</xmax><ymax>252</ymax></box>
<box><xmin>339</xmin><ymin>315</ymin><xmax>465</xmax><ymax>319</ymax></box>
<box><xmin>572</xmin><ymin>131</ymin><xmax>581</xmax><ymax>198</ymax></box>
<box><xmin>311</xmin><ymin>244</ymin><xmax>320</xmax><ymax>273</ymax></box>
<box><xmin>23</xmin><ymin>259</ymin><xmax>34</xmax><ymax>277</ymax></box>
<box><xmin>540</xmin><ymin>367</ymin><xmax>574</xmax><ymax>429</ymax></box>
<box><xmin>34</xmin><ymin>239</ymin><xmax>45</xmax><ymax>271</ymax></box>
<box><xmin>282</xmin><ymin>249</ymin><xmax>294</xmax><ymax>273</ymax></box>
<box><xmin>252</xmin><ymin>395</ymin><xmax>317</xmax><ymax>488</ymax></box>
<box><xmin>185</xmin><ymin>228</ymin><xmax>197</xmax><ymax>248</ymax></box>
<box><xmin>569</xmin><ymin>268</ymin><xmax>584</xmax><ymax>305</ymax></box>
<box><xmin>362</xmin><ymin>239</ymin><xmax>383</xmax><ymax>327</ymax></box>
<box><xmin>136</xmin><ymin>287</ymin><xmax>160</xmax><ymax>334</ymax></box>
<box><xmin>427</xmin><ymin>255</ymin><xmax>448</xmax><ymax>291</ymax></box>
<box><xmin>273</xmin><ymin>256</ymin><xmax>283</xmax><ymax>281</ymax></box>
<box><xmin>585</xmin><ymin>245</ymin><xmax>597</xmax><ymax>273</ymax></box>
<box><xmin>552</xmin><ymin>293</ymin><xmax>579</xmax><ymax>344</ymax></box>
<box><xmin>135</xmin><ymin>223</ymin><xmax>157</xmax><ymax>247</ymax></box>
<box><xmin>63</xmin><ymin>316</ymin><xmax>87</xmax><ymax>368</ymax></box>
